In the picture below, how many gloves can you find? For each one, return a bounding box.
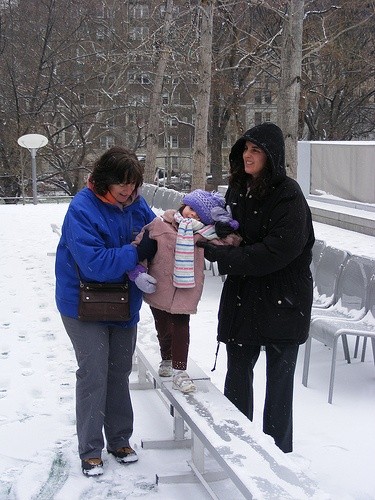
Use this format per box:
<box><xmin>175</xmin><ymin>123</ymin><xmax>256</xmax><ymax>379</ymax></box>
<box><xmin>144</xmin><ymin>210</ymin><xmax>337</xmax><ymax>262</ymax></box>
<box><xmin>129</xmin><ymin>264</ymin><xmax>157</xmax><ymax>294</ymax></box>
<box><xmin>136</xmin><ymin>228</ymin><xmax>158</xmax><ymax>262</ymax></box>
<box><xmin>196</xmin><ymin>241</ymin><xmax>236</xmax><ymax>262</ymax></box>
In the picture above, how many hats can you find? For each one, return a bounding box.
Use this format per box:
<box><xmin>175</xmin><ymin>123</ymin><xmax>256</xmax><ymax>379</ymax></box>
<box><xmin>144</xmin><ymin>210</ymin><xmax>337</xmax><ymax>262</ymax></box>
<box><xmin>183</xmin><ymin>189</ymin><xmax>239</xmax><ymax>230</ymax></box>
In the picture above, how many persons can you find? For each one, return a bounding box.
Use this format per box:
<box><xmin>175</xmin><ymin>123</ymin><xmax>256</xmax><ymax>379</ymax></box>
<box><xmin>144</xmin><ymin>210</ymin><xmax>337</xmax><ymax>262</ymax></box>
<box><xmin>197</xmin><ymin>123</ymin><xmax>314</xmax><ymax>453</ymax></box>
<box><xmin>54</xmin><ymin>146</ymin><xmax>163</xmax><ymax>478</ymax></box>
<box><xmin>126</xmin><ymin>190</ymin><xmax>241</xmax><ymax>393</ymax></box>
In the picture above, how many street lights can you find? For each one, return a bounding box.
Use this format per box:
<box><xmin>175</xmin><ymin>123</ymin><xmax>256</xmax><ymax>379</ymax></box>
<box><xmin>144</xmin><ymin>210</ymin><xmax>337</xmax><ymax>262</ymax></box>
<box><xmin>17</xmin><ymin>134</ymin><xmax>48</xmax><ymax>205</ymax></box>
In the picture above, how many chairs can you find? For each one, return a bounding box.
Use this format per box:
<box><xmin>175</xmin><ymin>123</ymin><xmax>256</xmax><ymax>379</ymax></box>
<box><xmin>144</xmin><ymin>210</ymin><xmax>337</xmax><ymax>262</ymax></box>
<box><xmin>142</xmin><ymin>183</ymin><xmax>375</xmax><ymax>404</ymax></box>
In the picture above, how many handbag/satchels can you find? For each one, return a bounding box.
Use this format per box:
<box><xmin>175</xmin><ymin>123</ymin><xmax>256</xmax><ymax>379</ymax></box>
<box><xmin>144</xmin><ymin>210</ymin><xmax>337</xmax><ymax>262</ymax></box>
<box><xmin>78</xmin><ymin>281</ymin><xmax>130</xmax><ymax>322</ymax></box>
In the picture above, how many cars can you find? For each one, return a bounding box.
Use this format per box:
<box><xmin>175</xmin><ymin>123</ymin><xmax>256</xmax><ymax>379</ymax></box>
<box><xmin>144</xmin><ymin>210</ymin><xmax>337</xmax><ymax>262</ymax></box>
<box><xmin>152</xmin><ymin>167</ymin><xmax>231</xmax><ymax>189</ymax></box>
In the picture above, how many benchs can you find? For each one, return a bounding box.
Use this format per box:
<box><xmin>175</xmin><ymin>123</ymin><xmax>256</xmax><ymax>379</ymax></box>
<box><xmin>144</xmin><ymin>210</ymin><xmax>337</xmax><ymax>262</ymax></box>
<box><xmin>128</xmin><ymin>335</ymin><xmax>324</xmax><ymax>500</ymax></box>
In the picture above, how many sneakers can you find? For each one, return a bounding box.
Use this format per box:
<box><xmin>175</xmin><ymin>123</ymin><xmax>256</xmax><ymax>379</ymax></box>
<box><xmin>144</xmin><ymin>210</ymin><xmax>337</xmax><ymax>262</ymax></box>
<box><xmin>81</xmin><ymin>458</ymin><xmax>103</xmax><ymax>477</ymax></box>
<box><xmin>158</xmin><ymin>360</ymin><xmax>172</xmax><ymax>377</ymax></box>
<box><xmin>106</xmin><ymin>441</ymin><xmax>138</xmax><ymax>464</ymax></box>
<box><xmin>172</xmin><ymin>371</ymin><xmax>197</xmax><ymax>393</ymax></box>
